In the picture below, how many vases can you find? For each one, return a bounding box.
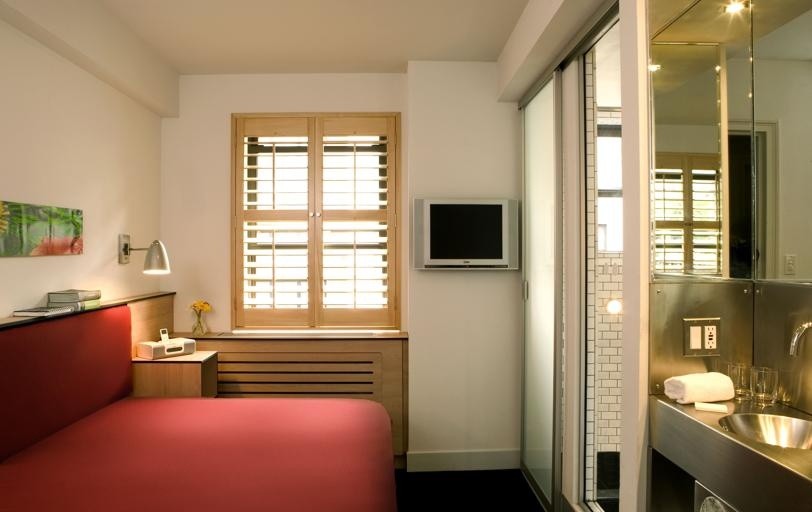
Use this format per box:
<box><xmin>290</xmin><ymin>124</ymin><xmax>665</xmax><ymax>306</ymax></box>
<box><xmin>192</xmin><ymin>318</ymin><xmax>208</xmax><ymax>338</ymax></box>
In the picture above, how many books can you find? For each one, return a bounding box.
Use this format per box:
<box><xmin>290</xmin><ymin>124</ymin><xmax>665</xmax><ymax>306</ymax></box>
<box><xmin>47</xmin><ymin>299</ymin><xmax>100</xmax><ymax>312</ymax></box>
<box><xmin>47</xmin><ymin>288</ymin><xmax>101</xmax><ymax>303</ymax></box>
<box><xmin>13</xmin><ymin>306</ymin><xmax>74</xmax><ymax>318</ymax></box>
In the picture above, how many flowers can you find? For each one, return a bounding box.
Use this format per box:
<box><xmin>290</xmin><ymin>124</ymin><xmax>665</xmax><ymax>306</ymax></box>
<box><xmin>189</xmin><ymin>299</ymin><xmax>211</xmax><ymax>335</ymax></box>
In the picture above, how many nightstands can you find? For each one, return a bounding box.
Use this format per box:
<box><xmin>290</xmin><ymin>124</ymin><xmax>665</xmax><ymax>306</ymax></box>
<box><xmin>130</xmin><ymin>350</ymin><xmax>218</xmax><ymax>398</ymax></box>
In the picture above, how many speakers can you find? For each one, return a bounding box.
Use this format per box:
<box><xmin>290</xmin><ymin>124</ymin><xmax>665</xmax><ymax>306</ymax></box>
<box><xmin>136</xmin><ymin>337</ymin><xmax>196</xmax><ymax>359</ymax></box>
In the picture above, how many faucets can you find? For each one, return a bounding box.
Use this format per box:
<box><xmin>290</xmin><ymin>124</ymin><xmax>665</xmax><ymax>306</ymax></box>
<box><xmin>789</xmin><ymin>322</ymin><xmax>812</xmax><ymax>357</ymax></box>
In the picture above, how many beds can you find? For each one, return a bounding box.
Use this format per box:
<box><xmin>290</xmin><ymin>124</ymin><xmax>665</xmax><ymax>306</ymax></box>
<box><xmin>0</xmin><ymin>301</ymin><xmax>397</xmax><ymax>512</ymax></box>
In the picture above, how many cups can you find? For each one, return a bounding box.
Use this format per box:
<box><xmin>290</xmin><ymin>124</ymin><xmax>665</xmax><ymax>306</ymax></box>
<box><xmin>728</xmin><ymin>362</ymin><xmax>753</xmax><ymax>401</ymax></box>
<box><xmin>750</xmin><ymin>366</ymin><xmax>778</xmax><ymax>407</ymax></box>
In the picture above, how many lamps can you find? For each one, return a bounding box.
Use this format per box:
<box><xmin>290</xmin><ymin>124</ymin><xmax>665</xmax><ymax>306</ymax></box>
<box><xmin>119</xmin><ymin>234</ymin><xmax>170</xmax><ymax>275</ymax></box>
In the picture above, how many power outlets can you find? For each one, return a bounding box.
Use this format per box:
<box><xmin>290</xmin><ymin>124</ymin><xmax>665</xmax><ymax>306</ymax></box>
<box><xmin>683</xmin><ymin>317</ymin><xmax>721</xmax><ymax>358</ymax></box>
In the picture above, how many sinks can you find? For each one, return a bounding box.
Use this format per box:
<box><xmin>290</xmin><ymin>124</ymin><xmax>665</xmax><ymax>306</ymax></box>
<box><xmin>719</xmin><ymin>413</ymin><xmax>812</xmax><ymax>449</ymax></box>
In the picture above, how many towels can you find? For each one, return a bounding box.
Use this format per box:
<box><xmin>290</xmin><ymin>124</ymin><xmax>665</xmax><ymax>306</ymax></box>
<box><xmin>664</xmin><ymin>372</ymin><xmax>735</xmax><ymax>404</ymax></box>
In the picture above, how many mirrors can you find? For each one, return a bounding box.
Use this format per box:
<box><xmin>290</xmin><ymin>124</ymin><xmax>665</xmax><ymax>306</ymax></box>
<box><xmin>650</xmin><ymin>0</ymin><xmax>812</xmax><ymax>288</ymax></box>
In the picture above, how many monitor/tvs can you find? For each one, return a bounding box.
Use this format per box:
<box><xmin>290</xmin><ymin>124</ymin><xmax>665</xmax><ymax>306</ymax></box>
<box><xmin>412</xmin><ymin>197</ymin><xmax>519</xmax><ymax>270</ymax></box>
